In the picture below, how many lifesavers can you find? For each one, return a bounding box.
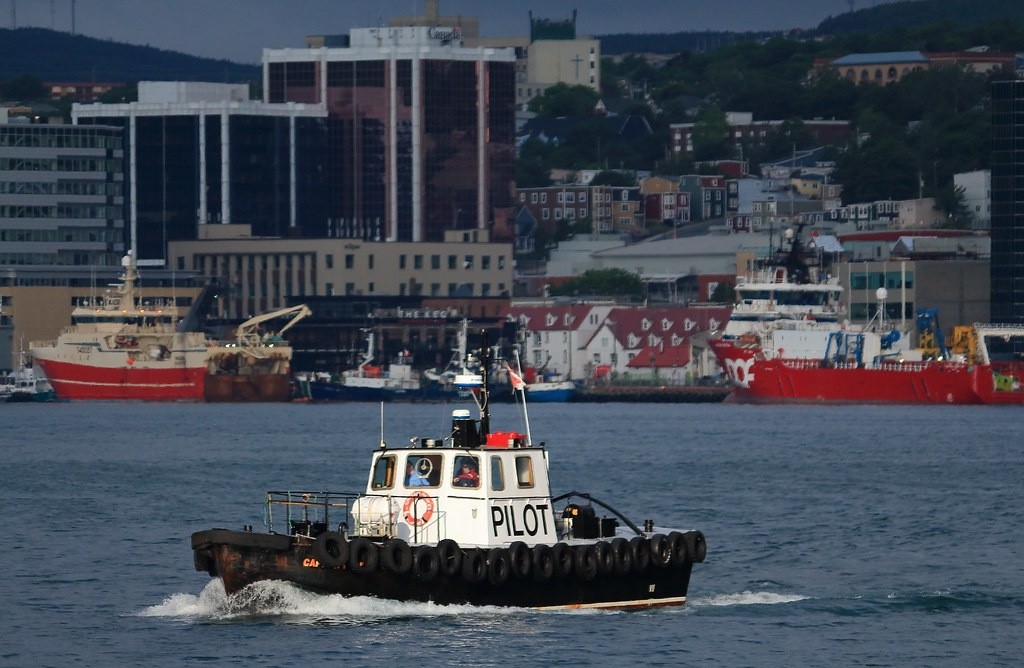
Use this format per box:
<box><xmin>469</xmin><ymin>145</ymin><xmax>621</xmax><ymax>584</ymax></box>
<box><xmin>315</xmin><ymin>530</ymin><xmax>707</xmax><ymax>585</ymax></box>
<box><xmin>210</xmin><ymin>340</ymin><xmax>217</xmax><ymax>348</ymax></box>
<box><xmin>848</xmin><ymin>358</ymin><xmax>855</xmax><ymax>369</ymax></box>
<box><xmin>404</xmin><ymin>491</ymin><xmax>433</xmax><ymax>526</ymax></box>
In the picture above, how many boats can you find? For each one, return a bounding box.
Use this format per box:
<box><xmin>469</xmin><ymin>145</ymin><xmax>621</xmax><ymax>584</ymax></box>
<box><xmin>19</xmin><ymin>248</ymin><xmax>307</xmax><ymax>405</ymax></box>
<box><xmin>296</xmin><ymin>315</ymin><xmax>737</xmax><ymax>404</ymax></box>
<box><xmin>180</xmin><ymin>341</ymin><xmax>711</xmax><ymax>611</ymax></box>
<box><xmin>707</xmin><ymin>217</ymin><xmax>1024</xmax><ymax>408</ymax></box>
<box><xmin>0</xmin><ymin>332</ymin><xmax>55</xmax><ymax>402</ymax></box>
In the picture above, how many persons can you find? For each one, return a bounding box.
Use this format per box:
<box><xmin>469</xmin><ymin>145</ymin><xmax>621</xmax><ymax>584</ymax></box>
<box><xmin>453</xmin><ymin>463</ymin><xmax>479</xmax><ymax>487</ymax></box>
<box><xmin>807</xmin><ymin>309</ymin><xmax>818</xmax><ymax>320</ymax></box>
<box><xmin>404</xmin><ymin>460</ymin><xmax>431</xmax><ymax>486</ymax></box>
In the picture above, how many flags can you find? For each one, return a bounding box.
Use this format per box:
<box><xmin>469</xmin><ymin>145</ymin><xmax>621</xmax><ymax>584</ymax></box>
<box><xmin>508</xmin><ymin>368</ymin><xmax>526</xmax><ymax>393</ymax></box>
